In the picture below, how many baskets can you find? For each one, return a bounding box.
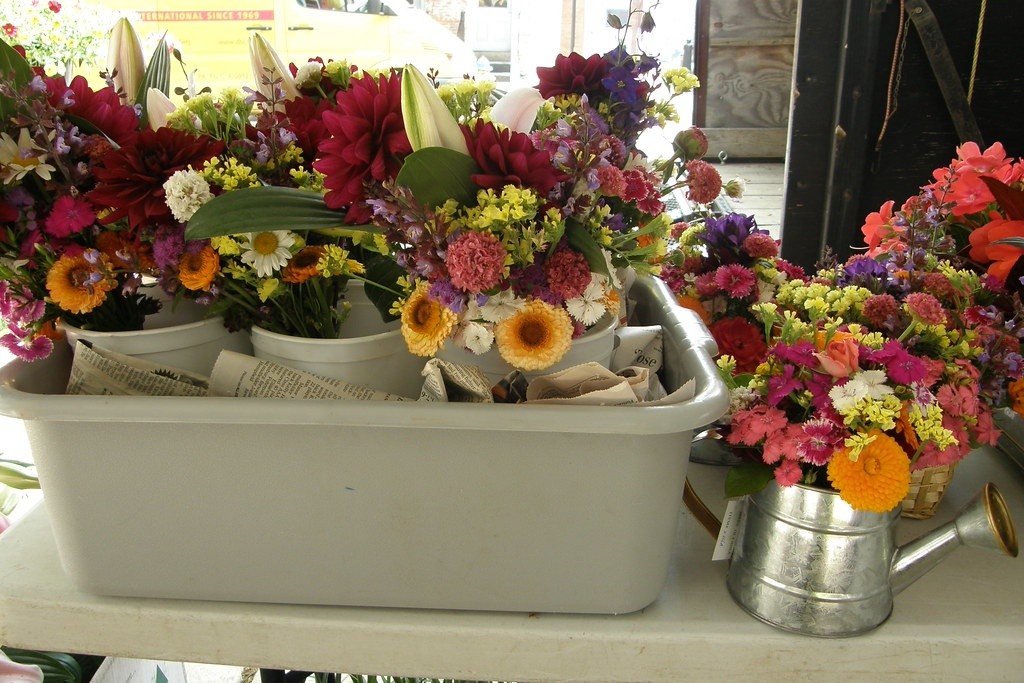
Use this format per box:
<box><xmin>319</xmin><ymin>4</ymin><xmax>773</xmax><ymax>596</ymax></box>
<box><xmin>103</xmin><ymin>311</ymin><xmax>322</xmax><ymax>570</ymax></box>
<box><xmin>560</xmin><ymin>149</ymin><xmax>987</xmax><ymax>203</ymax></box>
<box><xmin>901</xmin><ymin>464</ymin><xmax>954</xmax><ymax>519</ymax></box>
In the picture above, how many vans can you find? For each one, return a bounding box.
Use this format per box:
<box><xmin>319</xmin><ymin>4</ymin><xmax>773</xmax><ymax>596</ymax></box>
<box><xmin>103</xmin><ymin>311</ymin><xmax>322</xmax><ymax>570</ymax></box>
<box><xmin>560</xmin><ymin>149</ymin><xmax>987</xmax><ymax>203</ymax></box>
<box><xmin>73</xmin><ymin>0</ymin><xmax>475</xmax><ymax>108</ymax></box>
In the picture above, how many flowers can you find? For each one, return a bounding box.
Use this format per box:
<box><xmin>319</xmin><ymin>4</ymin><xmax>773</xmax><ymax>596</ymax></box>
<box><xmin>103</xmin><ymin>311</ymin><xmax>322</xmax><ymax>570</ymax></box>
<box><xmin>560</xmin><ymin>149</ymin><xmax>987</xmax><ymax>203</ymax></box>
<box><xmin>0</xmin><ymin>0</ymin><xmax>1023</xmax><ymax>514</ymax></box>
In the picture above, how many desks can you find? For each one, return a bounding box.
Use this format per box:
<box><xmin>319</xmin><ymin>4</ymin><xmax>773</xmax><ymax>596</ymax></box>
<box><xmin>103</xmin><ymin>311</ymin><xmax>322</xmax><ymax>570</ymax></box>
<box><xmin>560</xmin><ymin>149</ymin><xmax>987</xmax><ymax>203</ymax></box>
<box><xmin>1</xmin><ymin>443</ymin><xmax>1024</xmax><ymax>683</ymax></box>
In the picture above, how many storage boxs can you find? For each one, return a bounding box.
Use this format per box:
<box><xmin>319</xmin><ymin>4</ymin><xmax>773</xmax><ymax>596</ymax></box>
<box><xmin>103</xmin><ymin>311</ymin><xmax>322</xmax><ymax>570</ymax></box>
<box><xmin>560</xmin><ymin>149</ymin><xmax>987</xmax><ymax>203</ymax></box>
<box><xmin>0</xmin><ymin>274</ymin><xmax>731</xmax><ymax>615</ymax></box>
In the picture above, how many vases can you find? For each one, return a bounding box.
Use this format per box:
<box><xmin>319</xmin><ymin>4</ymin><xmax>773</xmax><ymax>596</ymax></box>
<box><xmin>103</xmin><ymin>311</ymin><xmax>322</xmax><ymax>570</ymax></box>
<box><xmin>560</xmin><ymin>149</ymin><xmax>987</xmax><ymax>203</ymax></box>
<box><xmin>56</xmin><ymin>281</ymin><xmax>250</xmax><ymax>379</ymax></box>
<box><xmin>250</xmin><ymin>275</ymin><xmax>428</xmax><ymax>400</ymax></box>
<box><xmin>431</xmin><ymin>309</ymin><xmax>619</xmax><ymax>388</ymax></box>
